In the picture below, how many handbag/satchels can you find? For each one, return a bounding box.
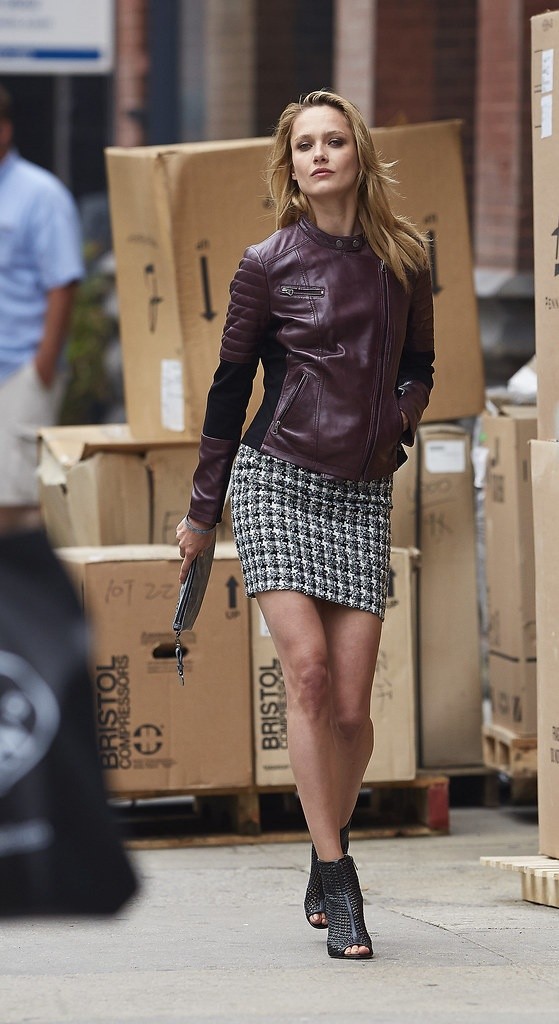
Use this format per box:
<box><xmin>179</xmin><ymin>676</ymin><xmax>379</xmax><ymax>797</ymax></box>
<box><xmin>172</xmin><ymin>523</ymin><xmax>217</xmax><ymax>686</ymax></box>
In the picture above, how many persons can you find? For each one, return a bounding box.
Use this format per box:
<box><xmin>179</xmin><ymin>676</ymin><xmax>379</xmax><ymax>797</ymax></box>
<box><xmin>0</xmin><ymin>106</ymin><xmax>86</xmax><ymax>535</ymax></box>
<box><xmin>173</xmin><ymin>86</ymin><xmax>435</xmax><ymax>963</ymax></box>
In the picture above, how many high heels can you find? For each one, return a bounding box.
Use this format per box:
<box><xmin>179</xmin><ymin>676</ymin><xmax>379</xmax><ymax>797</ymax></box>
<box><xmin>317</xmin><ymin>854</ymin><xmax>373</xmax><ymax>958</ymax></box>
<box><xmin>304</xmin><ymin>817</ymin><xmax>352</xmax><ymax>929</ymax></box>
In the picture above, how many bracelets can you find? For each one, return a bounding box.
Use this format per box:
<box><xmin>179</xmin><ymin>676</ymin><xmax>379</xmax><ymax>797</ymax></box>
<box><xmin>185</xmin><ymin>513</ymin><xmax>220</xmax><ymax>536</ymax></box>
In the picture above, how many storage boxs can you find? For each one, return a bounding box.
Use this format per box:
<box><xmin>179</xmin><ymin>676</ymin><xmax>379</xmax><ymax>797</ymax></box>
<box><xmin>37</xmin><ymin>10</ymin><xmax>559</xmax><ymax>859</ymax></box>
